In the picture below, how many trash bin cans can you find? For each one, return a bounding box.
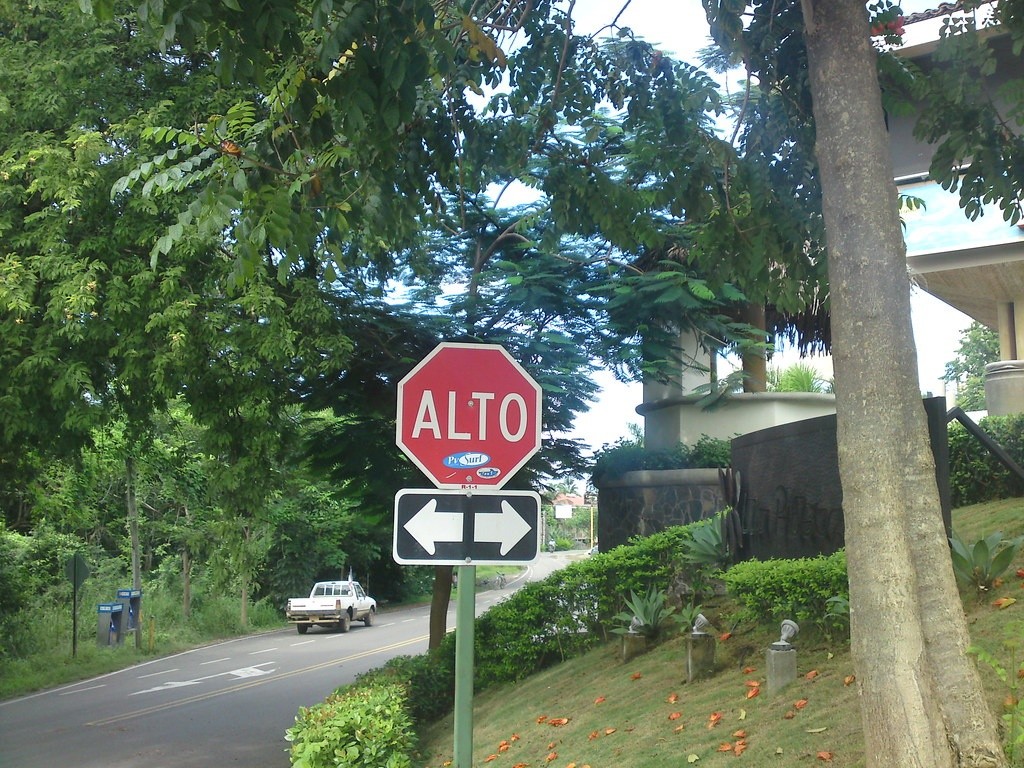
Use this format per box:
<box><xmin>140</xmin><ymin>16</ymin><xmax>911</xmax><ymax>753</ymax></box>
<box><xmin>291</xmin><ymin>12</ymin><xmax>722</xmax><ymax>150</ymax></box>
<box><xmin>96</xmin><ymin>602</ymin><xmax>124</xmax><ymax>648</ymax></box>
<box><xmin>117</xmin><ymin>589</ymin><xmax>142</xmax><ymax>635</ymax></box>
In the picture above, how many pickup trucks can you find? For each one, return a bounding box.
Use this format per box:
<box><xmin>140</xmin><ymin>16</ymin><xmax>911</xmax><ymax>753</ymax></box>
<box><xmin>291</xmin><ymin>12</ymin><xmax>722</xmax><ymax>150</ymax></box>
<box><xmin>286</xmin><ymin>581</ymin><xmax>378</xmax><ymax>633</ymax></box>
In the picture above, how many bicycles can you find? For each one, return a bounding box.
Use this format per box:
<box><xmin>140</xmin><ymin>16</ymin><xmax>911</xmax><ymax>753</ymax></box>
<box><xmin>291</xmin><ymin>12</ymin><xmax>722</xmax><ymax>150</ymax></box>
<box><xmin>496</xmin><ymin>571</ymin><xmax>506</xmax><ymax>590</ymax></box>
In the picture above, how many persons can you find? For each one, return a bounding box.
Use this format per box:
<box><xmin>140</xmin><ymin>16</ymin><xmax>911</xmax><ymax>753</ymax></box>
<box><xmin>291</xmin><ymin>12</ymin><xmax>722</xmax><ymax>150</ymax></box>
<box><xmin>549</xmin><ymin>539</ymin><xmax>556</xmax><ymax>548</ymax></box>
<box><xmin>452</xmin><ymin>571</ymin><xmax>458</xmax><ymax>589</ymax></box>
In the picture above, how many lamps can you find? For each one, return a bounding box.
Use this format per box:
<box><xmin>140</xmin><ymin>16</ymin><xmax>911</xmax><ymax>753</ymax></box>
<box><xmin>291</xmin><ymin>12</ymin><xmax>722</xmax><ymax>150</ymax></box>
<box><xmin>629</xmin><ymin>614</ymin><xmax>644</xmax><ymax>635</ymax></box>
<box><xmin>770</xmin><ymin>619</ymin><xmax>800</xmax><ymax>651</ymax></box>
<box><xmin>693</xmin><ymin>613</ymin><xmax>709</xmax><ymax>634</ymax></box>
